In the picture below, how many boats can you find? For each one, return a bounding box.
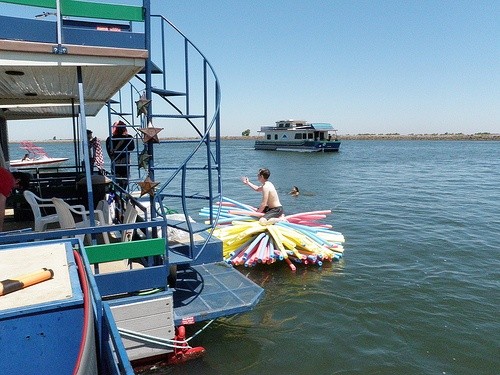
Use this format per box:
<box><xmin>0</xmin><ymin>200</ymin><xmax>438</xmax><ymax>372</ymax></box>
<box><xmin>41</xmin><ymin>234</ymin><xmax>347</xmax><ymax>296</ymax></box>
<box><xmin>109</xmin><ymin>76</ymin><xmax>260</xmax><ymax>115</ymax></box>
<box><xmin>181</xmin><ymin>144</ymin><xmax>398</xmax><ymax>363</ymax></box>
<box><xmin>254</xmin><ymin>120</ymin><xmax>341</xmax><ymax>153</ymax></box>
<box><xmin>10</xmin><ymin>140</ymin><xmax>69</xmax><ymax>168</ymax></box>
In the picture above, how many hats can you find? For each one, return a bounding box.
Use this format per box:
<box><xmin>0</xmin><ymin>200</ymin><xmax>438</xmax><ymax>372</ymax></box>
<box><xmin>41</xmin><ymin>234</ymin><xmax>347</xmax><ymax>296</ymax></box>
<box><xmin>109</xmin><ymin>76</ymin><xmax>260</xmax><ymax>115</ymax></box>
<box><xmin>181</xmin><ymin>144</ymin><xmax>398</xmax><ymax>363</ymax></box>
<box><xmin>112</xmin><ymin>120</ymin><xmax>128</xmax><ymax>135</ymax></box>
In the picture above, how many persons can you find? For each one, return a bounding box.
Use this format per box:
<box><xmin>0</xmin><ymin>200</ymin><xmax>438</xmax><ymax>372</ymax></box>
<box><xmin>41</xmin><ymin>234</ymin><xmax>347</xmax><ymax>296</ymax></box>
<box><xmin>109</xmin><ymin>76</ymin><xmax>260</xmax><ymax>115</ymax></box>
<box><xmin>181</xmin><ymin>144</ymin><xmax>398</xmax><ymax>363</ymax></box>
<box><xmin>287</xmin><ymin>185</ymin><xmax>300</xmax><ymax>198</ymax></box>
<box><xmin>240</xmin><ymin>168</ymin><xmax>290</xmax><ymax>226</ymax></box>
<box><xmin>0</xmin><ymin>165</ymin><xmax>31</xmax><ymax>233</ymax></box>
<box><xmin>21</xmin><ymin>153</ymin><xmax>31</xmax><ymax>162</ymax></box>
<box><xmin>81</xmin><ymin>129</ymin><xmax>99</xmax><ymax>172</ymax></box>
<box><xmin>105</xmin><ymin>120</ymin><xmax>136</xmax><ymax>192</ymax></box>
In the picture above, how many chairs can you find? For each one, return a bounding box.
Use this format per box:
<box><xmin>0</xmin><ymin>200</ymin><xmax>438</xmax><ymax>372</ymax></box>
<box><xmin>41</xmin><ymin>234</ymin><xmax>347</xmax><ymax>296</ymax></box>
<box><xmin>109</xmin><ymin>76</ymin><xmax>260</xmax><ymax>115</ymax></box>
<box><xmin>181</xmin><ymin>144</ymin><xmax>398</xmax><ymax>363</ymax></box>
<box><xmin>88</xmin><ymin>194</ymin><xmax>139</xmax><ymax>274</ymax></box>
<box><xmin>23</xmin><ymin>190</ymin><xmax>88</xmax><ymax>242</ymax></box>
<box><xmin>52</xmin><ymin>197</ymin><xmax>110</xmax><ymax>244</ymax></box>
